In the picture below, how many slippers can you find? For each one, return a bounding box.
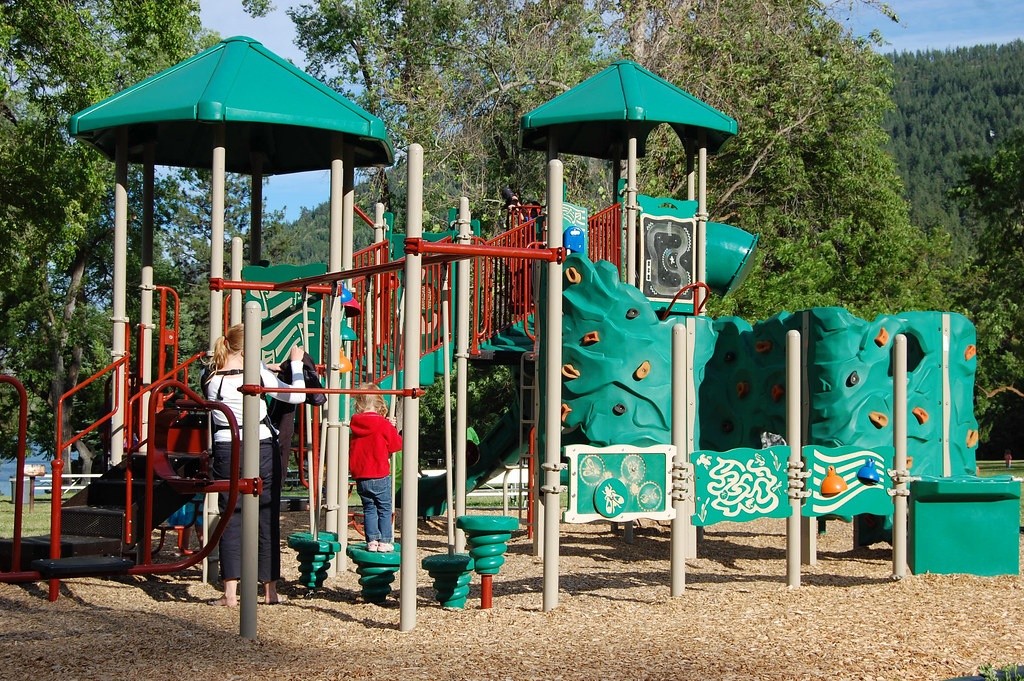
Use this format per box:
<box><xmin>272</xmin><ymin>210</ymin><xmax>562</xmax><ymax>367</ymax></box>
<box><xmin>206</xmin><ymin>598</ymin><xmax>237</xmax><ymax>607</ymax></box>
<box><xmin>265</xmin><ymin>594</ymin><xmax>289</xmax><ymax>605</ymax></box>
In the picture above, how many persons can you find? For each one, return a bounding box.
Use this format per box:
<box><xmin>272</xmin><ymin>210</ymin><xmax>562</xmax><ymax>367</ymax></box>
<box><xmin>394</xmin><ymin>429</ymin><xmax>428</xmax><ymax>497</ymax></box>
<box><xmin>348</xmin><ymin>383</ymin><xmax>403</xmax><ymax>552</ymax></box>
<box><xmin>466</xmin><ymin>416</ymin><xmax>480</xmax><ymax>445</ymax></box>
<box><xmin>200</xmin><ymin>323</ymin><xmax>306</xmax><ymax>606</ymax></box>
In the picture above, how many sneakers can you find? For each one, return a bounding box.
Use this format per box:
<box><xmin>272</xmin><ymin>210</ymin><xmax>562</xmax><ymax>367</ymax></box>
<box><xmin>378</xmin><ymin>542</ymin><xmax>394</xmax><ymax>552</ymax></box>
<box><xmin>365</xmin><ymin>541</ymin><xmax>378</xmax><ymax>552</ymax></box>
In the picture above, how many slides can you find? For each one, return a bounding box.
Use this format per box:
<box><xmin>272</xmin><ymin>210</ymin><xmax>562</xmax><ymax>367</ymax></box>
<box><xmin>395</xmin><ymin>360</ymin><xmax>534</xmax><ymax>517</ymax></box>
